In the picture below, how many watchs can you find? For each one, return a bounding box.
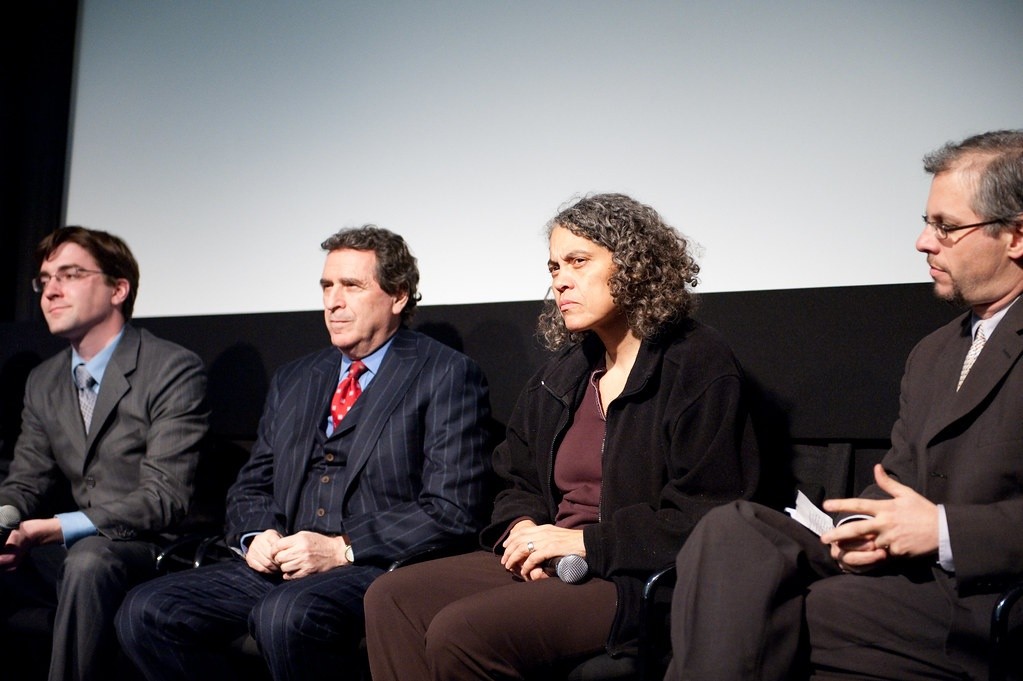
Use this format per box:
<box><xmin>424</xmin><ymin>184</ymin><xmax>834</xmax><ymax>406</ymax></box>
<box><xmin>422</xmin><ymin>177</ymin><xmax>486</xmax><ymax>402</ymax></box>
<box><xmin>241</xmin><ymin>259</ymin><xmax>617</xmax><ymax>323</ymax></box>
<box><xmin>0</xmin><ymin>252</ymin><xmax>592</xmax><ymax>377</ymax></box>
<box><xmin>342</xmin><ymin>533</ymin><xmax>354</xmax><ymax>565</ymax></box>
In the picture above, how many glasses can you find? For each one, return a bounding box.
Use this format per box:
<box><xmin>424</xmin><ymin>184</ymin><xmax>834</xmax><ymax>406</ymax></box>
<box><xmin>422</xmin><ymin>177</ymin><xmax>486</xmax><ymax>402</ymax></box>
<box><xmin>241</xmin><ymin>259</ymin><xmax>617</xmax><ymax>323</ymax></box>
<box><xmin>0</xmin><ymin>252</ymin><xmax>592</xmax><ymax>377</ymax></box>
<box><xmin>31</xmin><ymin>264</ymin><xmax>103</xmax><ymax>294</ymax></box>
<box><xmin>921</xmin><ymin>214</ymin><xmax>1007</xmax><ymax>241</ymax></box>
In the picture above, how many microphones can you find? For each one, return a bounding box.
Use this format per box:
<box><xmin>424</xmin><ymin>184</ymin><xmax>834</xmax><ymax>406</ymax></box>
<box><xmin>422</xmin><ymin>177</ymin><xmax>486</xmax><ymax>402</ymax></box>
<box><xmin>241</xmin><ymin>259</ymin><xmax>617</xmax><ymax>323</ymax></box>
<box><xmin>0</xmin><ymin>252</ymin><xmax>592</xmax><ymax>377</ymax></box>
<box><xmin>0</xmin><ymin>505</ymin><xmax>22</xmax><ymax>542</ymax></box>
<box><xmin>538</xmin><ymin>554</ymin><xmax>588</xmax><ymax>583</ymax></box>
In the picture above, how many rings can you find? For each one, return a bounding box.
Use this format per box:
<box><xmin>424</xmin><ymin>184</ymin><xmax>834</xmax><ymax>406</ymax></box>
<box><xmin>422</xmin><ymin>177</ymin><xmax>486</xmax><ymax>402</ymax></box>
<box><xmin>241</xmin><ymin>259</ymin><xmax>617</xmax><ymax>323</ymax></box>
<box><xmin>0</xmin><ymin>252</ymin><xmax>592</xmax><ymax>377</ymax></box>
<box><xmin>527</xmin><ymin>542</ymin><xmax>535</xmax><ymax>553</ymax></box>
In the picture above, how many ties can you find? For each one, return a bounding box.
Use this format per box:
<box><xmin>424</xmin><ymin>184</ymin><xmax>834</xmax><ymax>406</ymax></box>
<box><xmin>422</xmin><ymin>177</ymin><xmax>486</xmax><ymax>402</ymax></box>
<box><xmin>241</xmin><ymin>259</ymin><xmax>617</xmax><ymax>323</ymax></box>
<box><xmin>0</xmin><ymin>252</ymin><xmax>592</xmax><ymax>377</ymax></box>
<box><xmin>956</xmin><ymin>323</ymin><xmax>988</xmax><ymax>393</ymax></box>
<box><xmin>75</xmin><ymin>365</ymin><xmax>99</xmax><ymax>439</ymax></box>
<box><xmin>329</xmin><ymin>360</ymin><xmax>368</xmax><ymax>434</ymax></box>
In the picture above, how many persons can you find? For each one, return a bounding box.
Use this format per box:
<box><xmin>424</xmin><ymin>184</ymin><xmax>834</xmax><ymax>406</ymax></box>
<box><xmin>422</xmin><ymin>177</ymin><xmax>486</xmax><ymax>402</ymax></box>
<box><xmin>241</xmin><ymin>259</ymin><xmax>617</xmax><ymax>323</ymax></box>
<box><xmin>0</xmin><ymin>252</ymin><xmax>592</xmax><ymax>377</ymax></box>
<box><xmin>665</xmin><ymin>130</ymin><xmax>1023</xmax><ymax>681</ymax></box>
<box><xmin>363</xmin><ymin>195</ymin><xmax>759</xmax><ymax>680</ymax></box>
<box><xmin>0</xmin><ymin>226</ymin><xmax>211</xmax><ymax>681</ymax></box>
<box><xmin>114</xmin><ymin>229</ymin><xmax>492</xmax><ymax>681</ymax></box>
